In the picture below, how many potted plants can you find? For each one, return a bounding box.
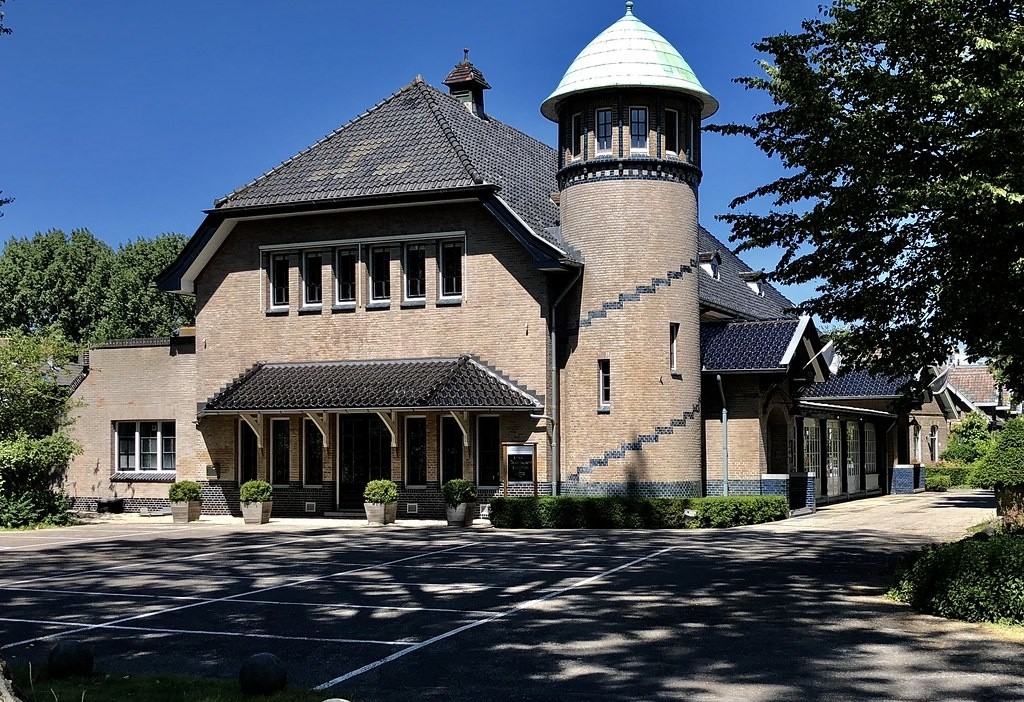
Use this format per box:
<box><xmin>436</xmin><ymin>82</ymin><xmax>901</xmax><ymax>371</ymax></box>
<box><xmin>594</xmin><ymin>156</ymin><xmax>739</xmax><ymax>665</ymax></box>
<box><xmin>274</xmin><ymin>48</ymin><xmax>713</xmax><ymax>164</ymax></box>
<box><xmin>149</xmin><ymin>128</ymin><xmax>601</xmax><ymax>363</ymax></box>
<box><xmin>168</xmin><ymin>479</ymin><xmax>203</xmax><ymax>524</ymax></box>
<box><xmin>440</xmin><ymin>479</ymin><xmax>479</xmax><ymax>527</ymax></box>
<box><xmin>239</xmin><ymin>479</ymin><xmax>274</xmax><ymax>524</ymax></box>
<box><xmin>363</xmin><ymin>479</ymin><xmax>398</xmax><ymax>525</ymax></box>
